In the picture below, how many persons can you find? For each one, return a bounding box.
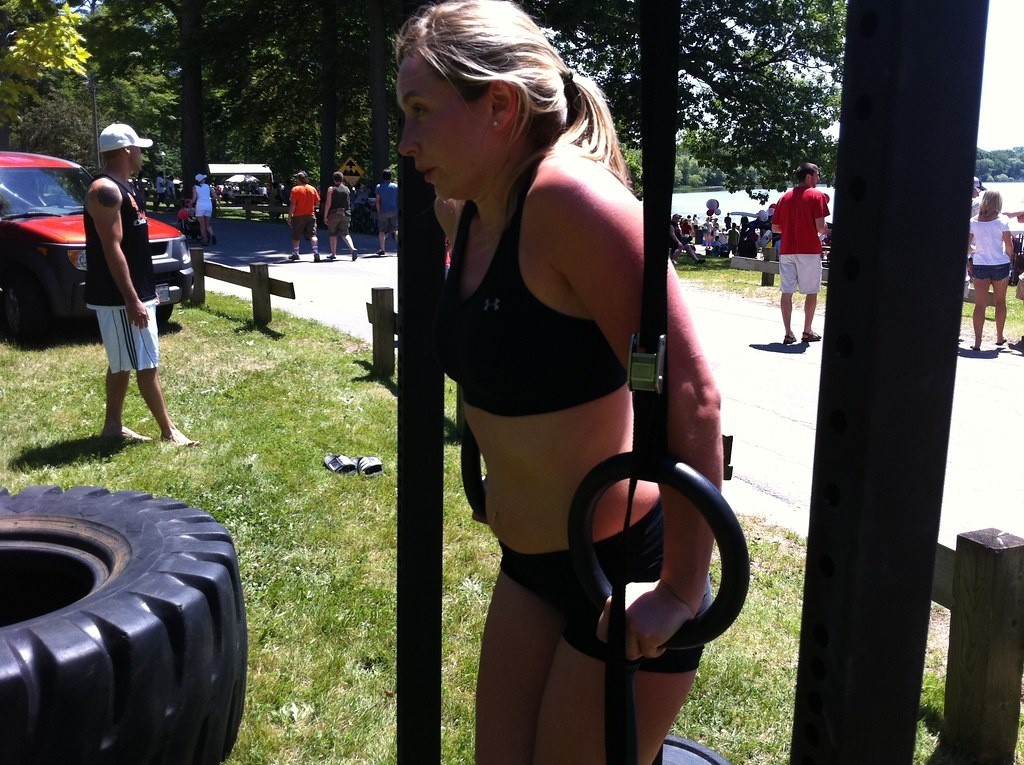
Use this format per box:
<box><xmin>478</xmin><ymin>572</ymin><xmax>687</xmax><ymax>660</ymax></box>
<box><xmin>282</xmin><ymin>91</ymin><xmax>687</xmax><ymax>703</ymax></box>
<box><xmin>770</xmin><ymin>163</ymin><xmax>830</xmax><ymax>344</ymax></box>
<box><xmin>184</xmin><ymin>174</ymin><xmax>217</xmax><ymax>246</ymax></box>
<box><xmin>967</xmin><ymin>176</ymin><xmax>1024</xmax><ymax>354</ymax></box>
<box><xmin>398</xmin><ymin>0</ymin><xmax>725</xmax><ymax>765</ymax></box>
<box><xmin>84</xmin><ymin>123</ymin><xmax>199</xmax><ymax>445</ymax></box>
<box><xmin>157</xmin><ymin>171</ymin><xmax>175</xmax><ymax>207</ymax></box>
<box><xmin>374</xmin><ymin>171</ymin><xmax>398</xmax><ymax>254</ymax></box>
<box><xmin>671</xmin><ymin>213</ymin><xmax>781</xmax><ymax>264</ymax></box>
<box><xmin>288</xmin><ymin>171</ymin><xmax>321</xmax><ymax>262</ymax></box>
<box><xmin>212</xmin><ymin>182</ymin><xmax>267</xmax><ymax>204</ymax></box>
<box><xmin>324</xmin><ymin>171</ymin><xmax>357</xmax><ymax>260</ymax></box>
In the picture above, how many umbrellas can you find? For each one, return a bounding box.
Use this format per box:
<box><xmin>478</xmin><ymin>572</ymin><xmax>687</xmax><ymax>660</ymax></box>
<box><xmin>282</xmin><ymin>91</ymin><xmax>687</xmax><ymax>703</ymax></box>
<box><xmin>226</xmin><ymin>174</ymin><xmax>259</xmax><ymax>182</ymax></box>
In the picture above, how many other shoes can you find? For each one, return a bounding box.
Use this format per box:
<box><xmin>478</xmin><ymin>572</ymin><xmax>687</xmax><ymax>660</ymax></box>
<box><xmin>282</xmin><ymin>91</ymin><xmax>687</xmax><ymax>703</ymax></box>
<box><xmin>376</xmin><ymin>249</ymin><xmax>385</xmax><ymax>255</ymax></box>
<box><xmin>288</xmin><ymin>251</ymin><xmax>300</xmax><ymax>260</ymax></box>
<box><xmin>352</xmin><ymin>249</ymin><xmax>358</xmax><ymax>261</ymax></box>
<box><xmin>211</xmin><ymin>235</ymin><xmax>217</xmax><ymax>244</ymax></box>
<box><xmin>326</xmin><ymin>255</ymin><xmax>336</xmax><ymax>259</ymax></box>
<box><xmin>314</xmin><ymin>254</ymin><xmax>320</xmax><ymax>262</ymax></box>
<box><xmin>200</xmin><ymin>241</ymin><xmax>209</xmax><ymax>246</ymax></box>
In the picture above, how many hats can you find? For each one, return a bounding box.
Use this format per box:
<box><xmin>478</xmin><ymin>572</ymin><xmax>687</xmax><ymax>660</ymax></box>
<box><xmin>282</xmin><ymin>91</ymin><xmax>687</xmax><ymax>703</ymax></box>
<box><xmin>769</xmin><ymin>203</ymin><xmax>777</xmax><ymax>215</ymax></box>
<box><xmin>98</xmin><ymin>123</ymin><xmax>154</xmax><ymax>154</ymax></box>
<box><xmin>195</xmin><ymin>174</ymin><xmax>207</xmax><ymax>183</ymax></box>
<box><xmin>756</xmin><ymin>210</ymin><xmax>768</xmax><ymax>222</ymax></box>
<box><xmin>973</xmin><ymin>176</ymin><xmax>987</xmax><ymax>191</ymax></box>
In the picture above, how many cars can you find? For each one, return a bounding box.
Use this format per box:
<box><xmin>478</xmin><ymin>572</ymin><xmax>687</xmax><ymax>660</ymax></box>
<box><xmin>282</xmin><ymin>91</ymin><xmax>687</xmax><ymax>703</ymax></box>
<box><xmin>0</xmin><ymin>151</ymin><xmax>194</xmax><ymax>347</ymax></box>
<box><xmin>350</xmin><ymin>185</ymin><xmax>379</xmax><ymax>233</ymax></box>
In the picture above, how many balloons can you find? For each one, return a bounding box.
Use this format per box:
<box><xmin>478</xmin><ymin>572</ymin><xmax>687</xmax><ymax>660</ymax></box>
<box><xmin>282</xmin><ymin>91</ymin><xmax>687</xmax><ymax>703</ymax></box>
<box><xmin>706</xmin><ymin>199</ymin><xmax>720</xmax><ymax>216</ymax></box>
<box><xmin>757</xmin><ymin>204</ymin><xmax>776</xmax><ymax>220</ymax></box>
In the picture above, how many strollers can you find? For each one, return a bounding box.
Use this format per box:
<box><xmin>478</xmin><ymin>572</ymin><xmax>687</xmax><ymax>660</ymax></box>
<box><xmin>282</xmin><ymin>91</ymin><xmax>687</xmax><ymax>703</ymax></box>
<box><xmin>174</xmin><ymin>198</ymin><xmax>201</xmax><ymax>240</ymax></box>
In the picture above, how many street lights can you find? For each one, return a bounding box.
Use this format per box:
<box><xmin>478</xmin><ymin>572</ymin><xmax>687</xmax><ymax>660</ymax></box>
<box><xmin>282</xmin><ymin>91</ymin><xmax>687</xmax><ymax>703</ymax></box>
<box><xmin>161</xmin><ymin>152</ymin><xmax>166</xmax><ymax>180</ymax></box>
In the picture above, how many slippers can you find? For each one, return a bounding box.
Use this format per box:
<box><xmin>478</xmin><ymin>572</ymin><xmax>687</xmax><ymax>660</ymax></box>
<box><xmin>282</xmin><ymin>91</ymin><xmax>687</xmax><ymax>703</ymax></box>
<box><xmin>996</xmin><ymin>337</ymin><xmax>1007</xmax><ymax>345</ymax></box>
<box><xmin>970</xmin><ymin>345</ymin><xmax>980</xmax><ymax>351</ymax></box>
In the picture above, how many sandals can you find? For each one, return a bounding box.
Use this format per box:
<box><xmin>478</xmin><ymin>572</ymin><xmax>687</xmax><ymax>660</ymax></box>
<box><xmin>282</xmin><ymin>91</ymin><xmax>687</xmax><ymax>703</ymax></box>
<box><xmin>783</xmin><ymin>334</ymin><xmax>797</xmax><ymax>345</ymax></box>
<box><xmin>800</xmin><ymin>331</ymin><xmax>822</xmax><ymax>342</ymax></box>
